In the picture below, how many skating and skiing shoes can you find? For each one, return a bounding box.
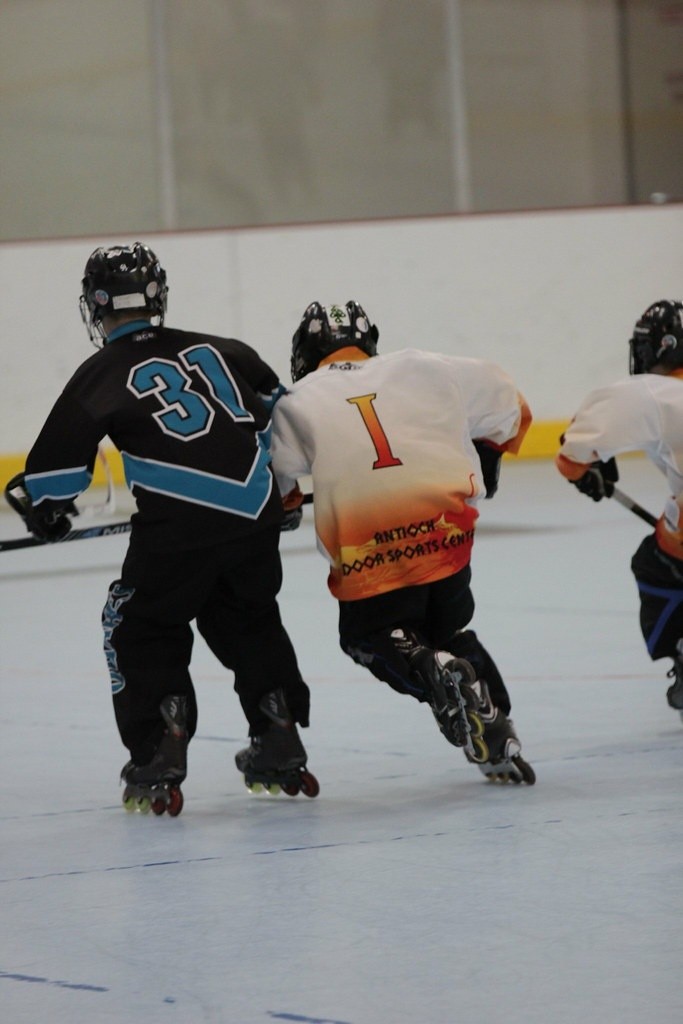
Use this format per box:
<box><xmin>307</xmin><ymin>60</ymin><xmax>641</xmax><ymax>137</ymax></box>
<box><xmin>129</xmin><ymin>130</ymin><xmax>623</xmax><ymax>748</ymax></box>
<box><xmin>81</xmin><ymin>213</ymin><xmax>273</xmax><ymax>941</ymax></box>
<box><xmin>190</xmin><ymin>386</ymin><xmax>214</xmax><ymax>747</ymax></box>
<box><xmin>666</xmin><ymin>638</ymin><xmax>683</xmax><ymax>709</ymax></box>
<box><xmin>469</xmin><ymin>679</ymin><xmax>537</xmax><ymax>784</ymax></box>
<box><xmin>120</xmin><ymin>694</ymin><xmax>197</xmax><ymax>817</ymax></box>
<box><xmin>235</xmin><ymin>689</ymin><xmax>320</xmax><ymax>798</ymax></box>
<box><xmin>373</xmin><ymin>620</ymin><xmax>489</xmax><ymax>764</ymax></box>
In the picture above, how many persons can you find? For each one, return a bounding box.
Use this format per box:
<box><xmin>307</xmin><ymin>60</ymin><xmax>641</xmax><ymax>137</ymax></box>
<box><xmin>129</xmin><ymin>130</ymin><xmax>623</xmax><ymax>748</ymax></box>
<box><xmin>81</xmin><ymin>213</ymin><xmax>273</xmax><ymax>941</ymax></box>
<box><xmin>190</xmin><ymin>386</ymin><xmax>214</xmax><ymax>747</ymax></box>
<box><xmin>268</xmin><ymin>299</ymin><xmax>535</xmax><ymax>787</ymax></box>
<box><xmin>4</xmin><ymin>243</ymin><xmax>319</xmax><ymax>816</ymax></box>
<box><xmin>558</xmin><ymin>300</ymin><xmax>682</xmax><ymax>710</ymax></box>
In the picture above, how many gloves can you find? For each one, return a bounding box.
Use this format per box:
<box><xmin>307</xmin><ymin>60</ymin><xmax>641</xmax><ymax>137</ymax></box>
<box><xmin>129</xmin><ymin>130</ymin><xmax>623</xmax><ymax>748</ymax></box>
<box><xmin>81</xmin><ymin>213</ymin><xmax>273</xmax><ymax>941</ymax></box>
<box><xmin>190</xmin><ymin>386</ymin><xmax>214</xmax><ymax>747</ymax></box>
<box><xmin>5</xmin><ymin>471</ymin><xmax>80</xmax><ymax>543</ymax></box>
<box><xmin>569</xmin><ymin>460</ymin><xmax>608</xmax><ymax>501</ymax></box>
<box><xmin>280</xmin><ymin>505</ymin><xmax>302</xmax><ymax>530</ymax></box>
<box><xmin>472</xmin><ymin>438</ymin><xmax>508</xmax><ymax>498</ymax></box>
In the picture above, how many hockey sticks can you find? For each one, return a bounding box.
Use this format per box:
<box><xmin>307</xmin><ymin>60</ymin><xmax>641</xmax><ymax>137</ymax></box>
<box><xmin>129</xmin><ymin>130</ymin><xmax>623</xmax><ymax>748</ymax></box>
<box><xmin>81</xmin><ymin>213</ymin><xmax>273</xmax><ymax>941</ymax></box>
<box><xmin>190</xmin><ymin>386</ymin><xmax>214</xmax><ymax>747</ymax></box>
<box><xmin>604</xmin><ymin>481</ymin><xmax>659</xmax><ymax>528</ymax></box>
<box><xmin>0</xmin><ymin>493</ymin><xmax>315</xmax><ymax>553</ymax></box>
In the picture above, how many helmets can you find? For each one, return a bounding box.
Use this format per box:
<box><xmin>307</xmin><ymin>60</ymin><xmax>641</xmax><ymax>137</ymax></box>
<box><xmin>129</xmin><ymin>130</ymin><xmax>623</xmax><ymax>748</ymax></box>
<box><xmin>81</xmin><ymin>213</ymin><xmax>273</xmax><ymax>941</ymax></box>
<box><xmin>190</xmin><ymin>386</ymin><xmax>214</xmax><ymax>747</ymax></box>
<box><xmin>291</xmin><ymin>301</ymin><xmax>379</xmax><ymax>382</ymax></box>
<box><xmin>629</xmin><ymin>300</ymin><xmax>683</xmax><ymax>378</ymax></box>
<box><xmin>79</xmin><ymin>242</ymin><xmax>169</xmax><ymax>350</ymax></box>
